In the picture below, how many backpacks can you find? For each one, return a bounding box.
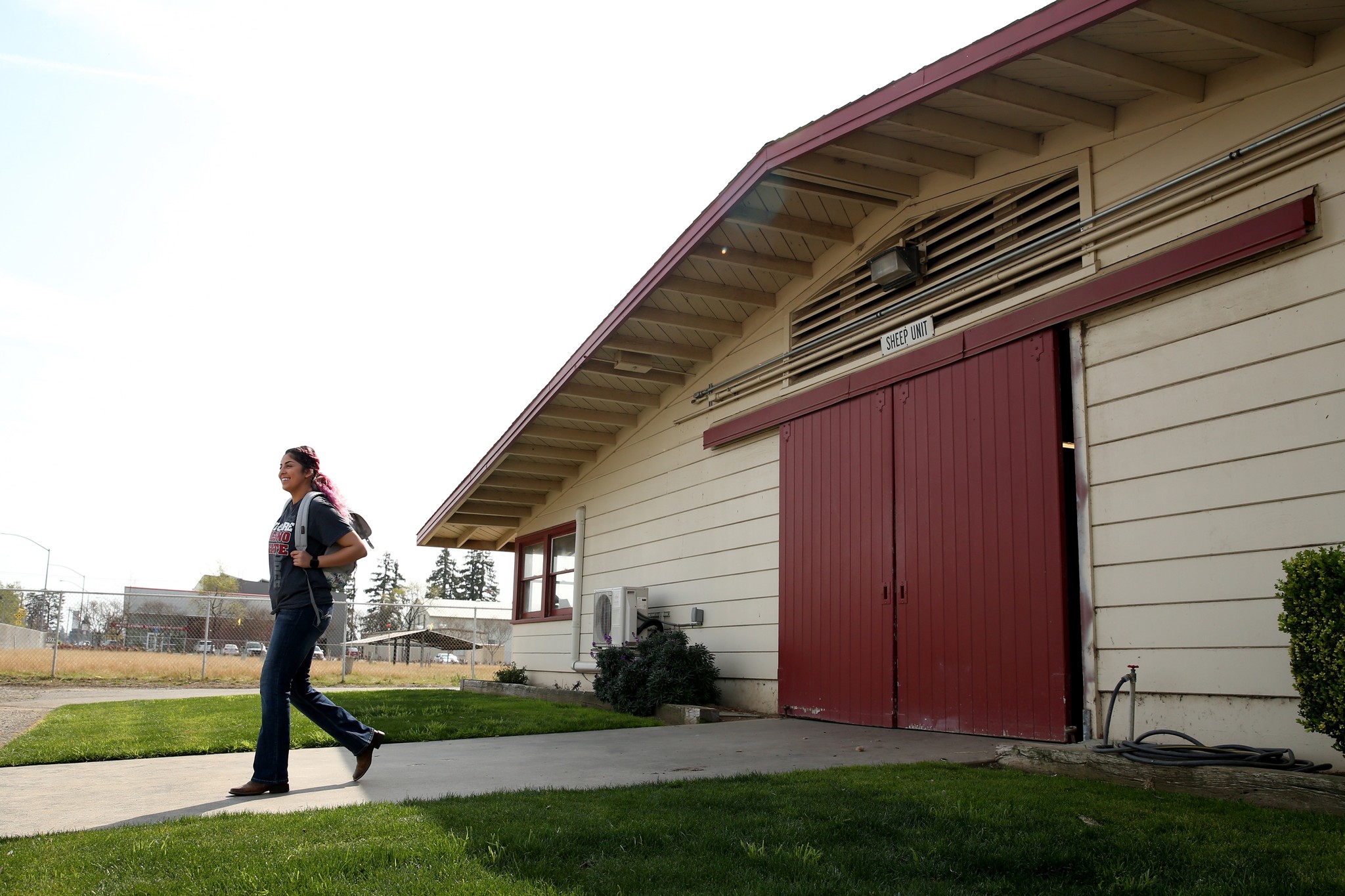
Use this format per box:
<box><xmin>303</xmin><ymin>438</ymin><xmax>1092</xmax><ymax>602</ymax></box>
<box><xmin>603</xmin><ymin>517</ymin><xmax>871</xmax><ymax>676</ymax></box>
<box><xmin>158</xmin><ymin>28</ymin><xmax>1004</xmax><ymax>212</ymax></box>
<box><xmin>282</xmin><ymin>491</ymin><xmax>375</xmax><ymax>589</ymax></box>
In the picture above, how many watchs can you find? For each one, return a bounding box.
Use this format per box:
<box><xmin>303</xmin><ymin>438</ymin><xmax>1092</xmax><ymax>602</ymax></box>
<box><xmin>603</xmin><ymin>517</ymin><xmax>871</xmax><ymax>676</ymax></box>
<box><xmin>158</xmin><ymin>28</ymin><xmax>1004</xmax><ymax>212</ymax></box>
<box><xmin>310</xmin><ymin>555</ymin><xmax>319</xmax><ymax>568</ymax></box>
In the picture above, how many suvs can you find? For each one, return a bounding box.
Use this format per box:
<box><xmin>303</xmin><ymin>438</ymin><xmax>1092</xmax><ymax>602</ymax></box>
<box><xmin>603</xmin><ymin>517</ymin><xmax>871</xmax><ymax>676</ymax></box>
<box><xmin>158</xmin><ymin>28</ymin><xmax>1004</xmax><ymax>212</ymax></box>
<box><xmin>192</xmin><ymin>640</ymin><xmax>215</xmax><ymax>654</ymax></box>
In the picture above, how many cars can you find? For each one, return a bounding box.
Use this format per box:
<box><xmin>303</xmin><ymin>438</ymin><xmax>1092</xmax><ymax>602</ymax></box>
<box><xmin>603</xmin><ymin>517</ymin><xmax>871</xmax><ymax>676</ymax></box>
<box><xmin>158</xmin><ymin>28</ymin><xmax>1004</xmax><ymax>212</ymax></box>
<box><xmin>222</xmin><ymin>644</ymin><xmax>240</xmax><ymax>656</ymax></box>
<box><xmin>100</xmin><ymin>640</ymin><xmax>122</xmax><ymax>650</ymax></box>
<box><xmin>346</xmin><ymin>647</ymin><xmax>361</xmax><ymax>659</ymax></box>
<box><xmin>312</xmin><ymin>646</ymin><xmax>325</xmax><ymax>659</ymax></box>
<box><xmin>243</xmin><ymin>641</ymin><xmax>265</xmax><ymax>656</ymax></box>
<box><xmin>435</xmin><ymin>653</ymin><xmax>459</xmax><ymax>664</ymax></box>
<box><xmin>75</xmin><ymin>640</ymin><xmax>93</xmax><ymax>647</ymax></box>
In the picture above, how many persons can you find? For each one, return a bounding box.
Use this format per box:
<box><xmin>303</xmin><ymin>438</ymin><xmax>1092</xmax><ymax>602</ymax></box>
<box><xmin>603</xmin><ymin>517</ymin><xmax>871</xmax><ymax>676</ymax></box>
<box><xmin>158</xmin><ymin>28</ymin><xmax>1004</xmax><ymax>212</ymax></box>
<box><xmin>227</xmin><ymin>447</ymin><xmax>384</xmax><ymax>796</ymax></box>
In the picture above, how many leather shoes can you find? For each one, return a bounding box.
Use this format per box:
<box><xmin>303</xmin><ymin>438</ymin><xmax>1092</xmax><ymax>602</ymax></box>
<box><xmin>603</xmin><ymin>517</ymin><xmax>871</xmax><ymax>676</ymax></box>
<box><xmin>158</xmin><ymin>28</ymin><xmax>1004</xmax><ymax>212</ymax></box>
<box><xmin>352</xmin><ymin>729</ymin><xmax>384</xmax><ymax>781</ymax></box>
<box><xmin>228</xmin><ymin>780</ymin><xmax>290</xmax><ymax>796</ymax></box>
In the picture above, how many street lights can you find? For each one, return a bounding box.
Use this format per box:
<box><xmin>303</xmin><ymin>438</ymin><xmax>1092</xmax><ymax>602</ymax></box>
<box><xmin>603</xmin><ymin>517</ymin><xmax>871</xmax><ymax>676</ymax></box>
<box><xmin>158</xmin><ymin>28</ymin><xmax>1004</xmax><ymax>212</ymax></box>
<box><xmin>59</xmin><ymin>580</ymin><xmax>90</xmax><ymax>622</ymax></box>
